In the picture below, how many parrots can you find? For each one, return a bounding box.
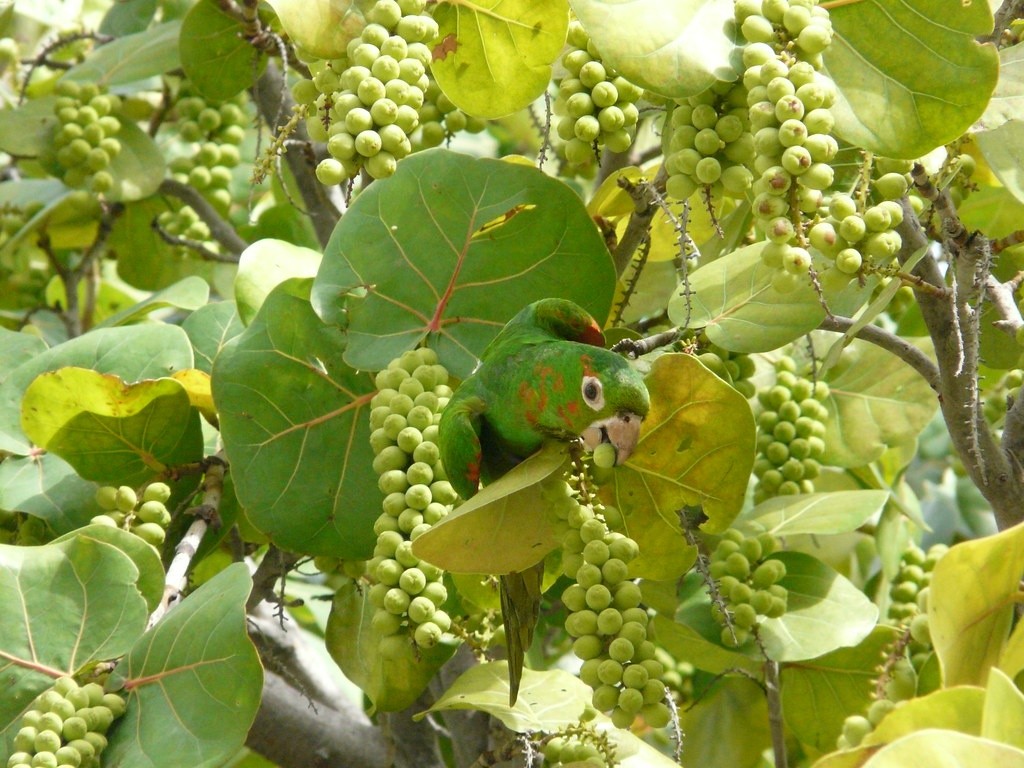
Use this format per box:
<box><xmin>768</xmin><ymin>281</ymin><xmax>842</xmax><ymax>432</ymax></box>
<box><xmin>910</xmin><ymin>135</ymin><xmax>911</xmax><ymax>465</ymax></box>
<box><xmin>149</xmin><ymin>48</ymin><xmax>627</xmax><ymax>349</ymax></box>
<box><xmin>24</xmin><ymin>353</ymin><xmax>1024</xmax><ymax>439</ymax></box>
<box><xmin>439</xmin><ymin>298</ymin><xmax>652</xmax><ymax>707</ymax></box>
